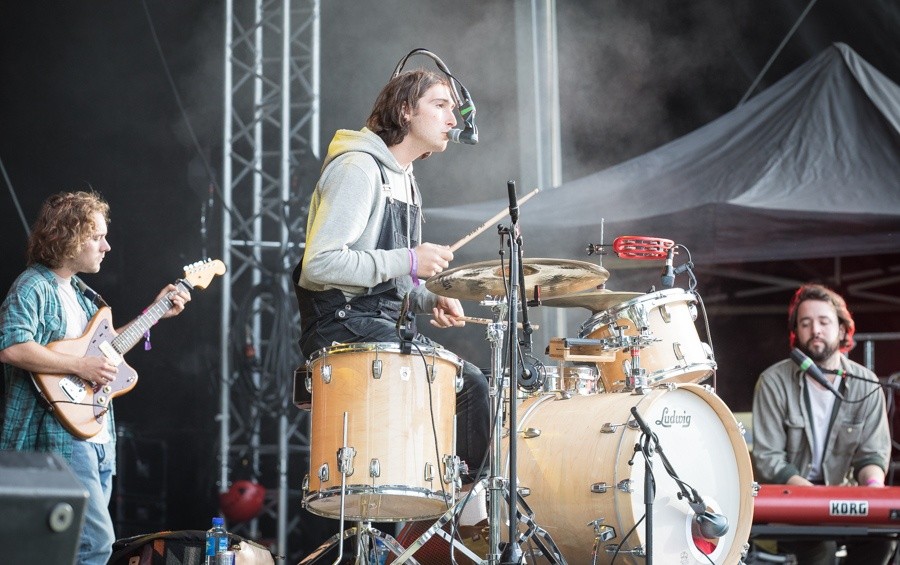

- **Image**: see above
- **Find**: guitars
[28,258,225,439]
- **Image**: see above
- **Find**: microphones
[446,101,479,146]
[660,247,676,287]
[790,348,844,400]
[517,354,547,394]
[692,514,730,539]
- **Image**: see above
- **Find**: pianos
[740,485,900,535]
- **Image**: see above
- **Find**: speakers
[0,450,91,564]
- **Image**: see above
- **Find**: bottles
[206,517,228,565]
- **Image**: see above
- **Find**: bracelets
[136,315,152,351]
[143,309,158,326]
[407,249,420,288]
[864,479,884,487]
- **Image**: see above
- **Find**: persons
[290,70,519,565]
[752,285,897,564]
[0,193,195,565]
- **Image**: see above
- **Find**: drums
[301,341,463,522]
[492,387,759,565]
[578,287,718,395]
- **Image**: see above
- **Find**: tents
[417,40,900,478]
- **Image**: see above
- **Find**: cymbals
[425,257,609,302]
[529,291,647,311]
[613,237,674,261]
